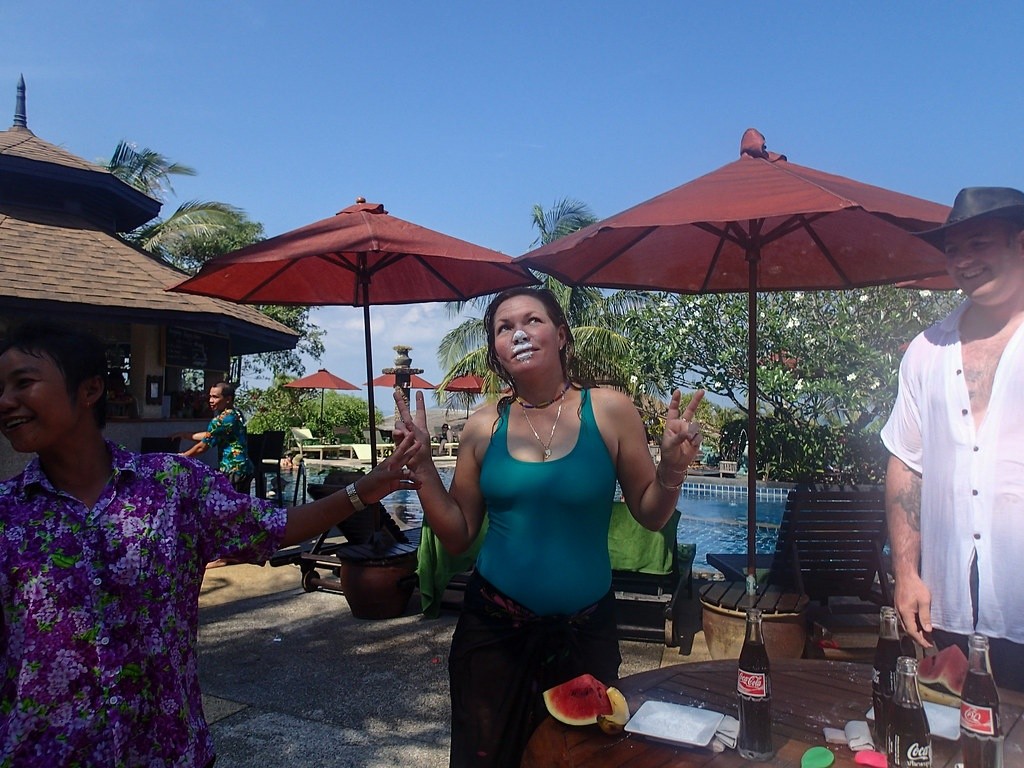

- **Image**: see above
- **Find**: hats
[909,187,1023,254]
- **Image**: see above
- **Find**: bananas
[597,686,630,735]
[918,685,962,710]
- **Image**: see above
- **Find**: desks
[520,649,1024,768]
[335,541,419,618]
[697,582,810,663]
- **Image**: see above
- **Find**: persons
[0,315,422,768]
[169,380,254,568]
[391,286,705,768]
[279,450,309,474]
[879,186,1024,693]
[431,423,465,455]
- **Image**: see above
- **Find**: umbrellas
[365,373,438,390]
[514,127,953,599]
[167,197,546,469]
[444,370,484,420]
[281,368,361,423]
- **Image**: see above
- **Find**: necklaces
[519,382,567,458]
[514,379,572,408]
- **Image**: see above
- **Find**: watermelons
[914,645,967,698]
[543,674,612,730]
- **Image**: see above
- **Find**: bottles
[746,568,756,595]
[885,656,932,768]
[960,634,1004,768]
[873,605,903,752]
[737,608,773,761]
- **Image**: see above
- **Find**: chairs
[299,469,422,592]
[288,425,459,461]
[607,499,696,648]
[705,482,895,664]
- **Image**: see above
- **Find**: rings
[681,416,692,424]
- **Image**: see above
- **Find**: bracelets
[656,468,687,491]
[659,459,689,474]
[345,482,366,513]
